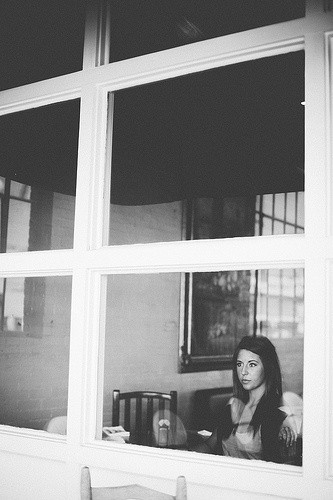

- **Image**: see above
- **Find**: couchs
[193,386,236,439]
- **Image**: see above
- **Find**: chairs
[111,387,178,432]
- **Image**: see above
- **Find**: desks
[125,427,222,456]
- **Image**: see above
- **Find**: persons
[190,334,302,467]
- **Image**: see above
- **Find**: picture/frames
[176,189,262,376]
